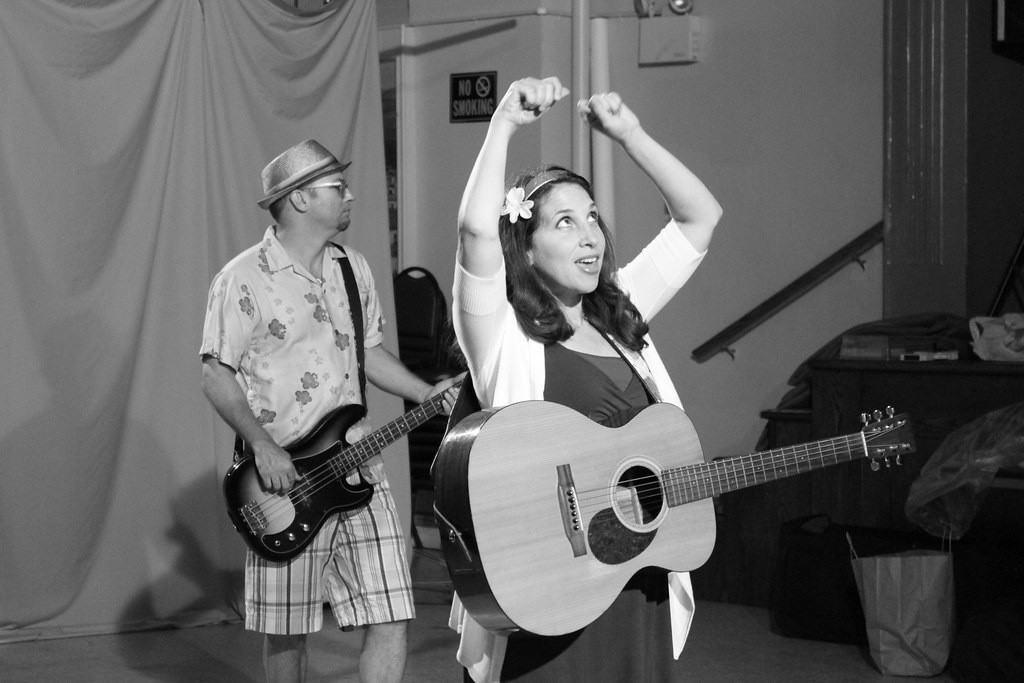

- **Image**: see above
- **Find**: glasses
[296,179,348,193]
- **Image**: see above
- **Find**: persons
[199,136,472,683]
[449,74,725,683]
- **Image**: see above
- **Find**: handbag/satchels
[846,522,955,677]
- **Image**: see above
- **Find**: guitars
[434,399,917,638]
[222,379,464,563]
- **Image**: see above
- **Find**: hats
[257,138,353,210]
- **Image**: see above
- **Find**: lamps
[634,0,701,65]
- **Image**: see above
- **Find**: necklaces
[562,311,583,328]
